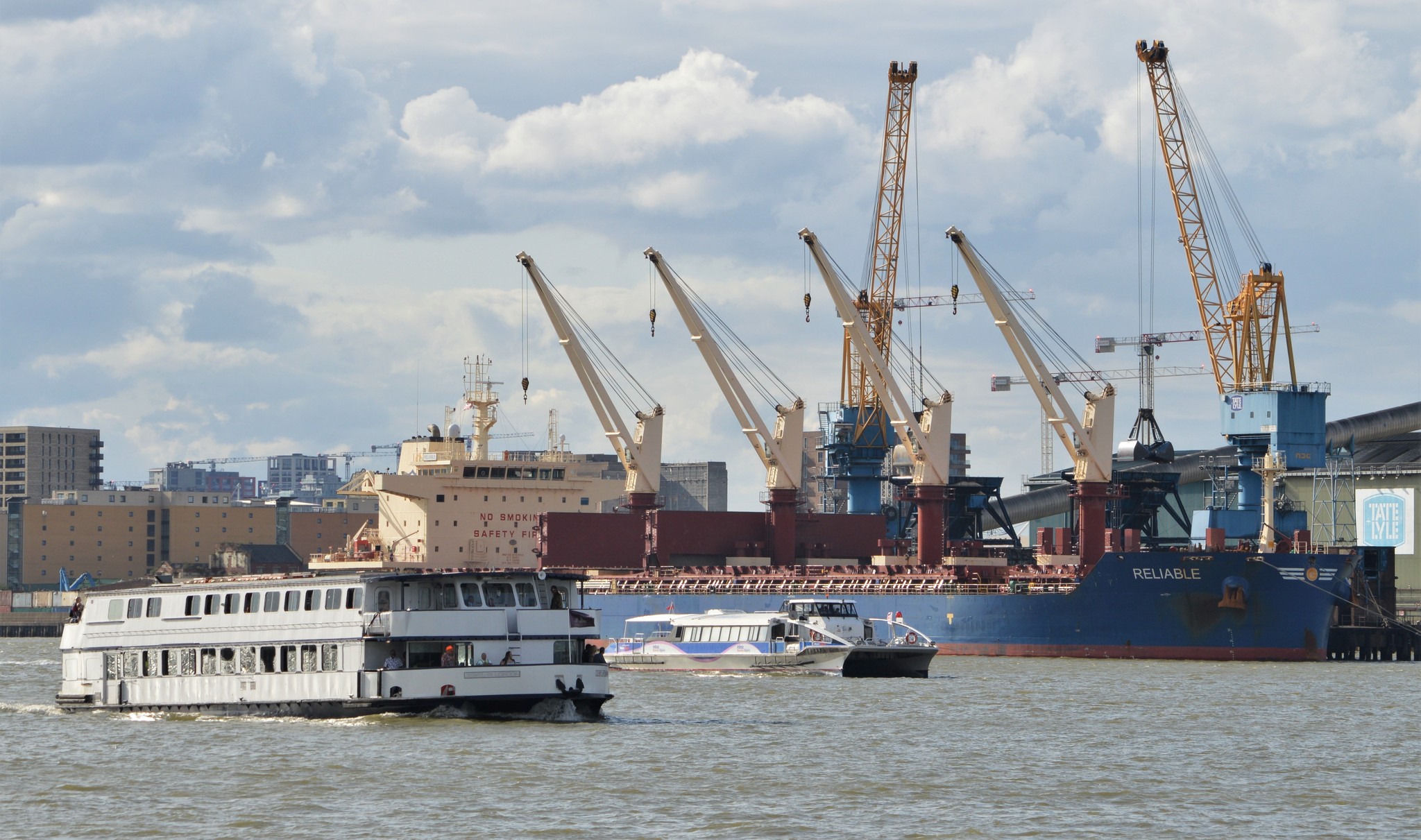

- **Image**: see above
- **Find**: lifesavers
[813,631,823,642]
[906,632,917,644]
[810,629,817,637]
[1000,586,1005,592]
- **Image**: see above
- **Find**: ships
[305,39,1370,663]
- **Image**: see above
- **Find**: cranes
[1136,40,1330,546]
[820,58,969,546]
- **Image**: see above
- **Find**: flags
[666,605,674,610]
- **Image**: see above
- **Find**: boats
[55,568,614,710]
[604,598,939,679]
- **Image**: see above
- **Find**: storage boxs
[532,513,886,568]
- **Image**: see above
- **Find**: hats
[446,646,452,651]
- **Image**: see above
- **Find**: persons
[441,646,459,667]
[550,585,563,610]
[466,596,482,607]
[477,653,493,666]
[819,606,840,614]
[500,651,516,665]
[69,597,84,620]
[582,644,610,666]
[383,650,403,670]
[496,588,509,607]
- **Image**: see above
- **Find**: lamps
[931,641,935,646]
[856,640,861,645]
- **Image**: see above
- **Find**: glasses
[391,654,396,656]
[551,589,555,591]
